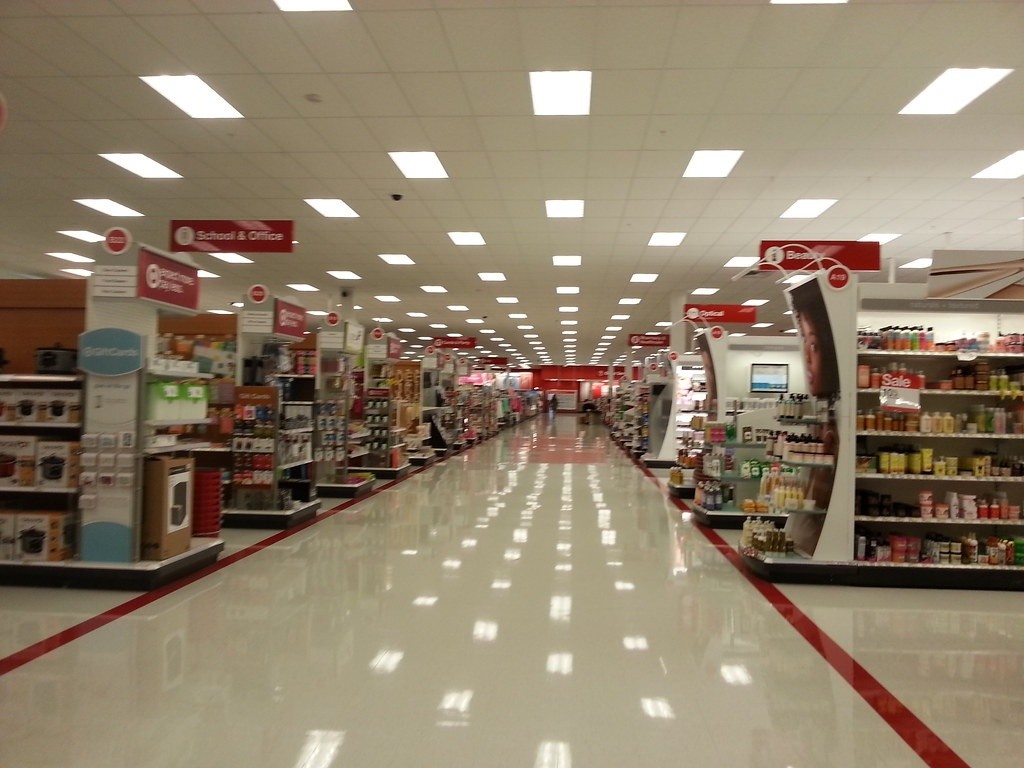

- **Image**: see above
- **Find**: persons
[583,399,595,421]
[788,277,839,396]
[699,351,717,411]
[550,395,558,418]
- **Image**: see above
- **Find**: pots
[38,454,65,480]
[48,400,65,417]
[1,453,16,477]
[17,400,33,415]
[19,530,44,553]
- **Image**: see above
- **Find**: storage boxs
[278,433,313,465]
[274,373,316,405]
[0,513,76,562]
[0,435,84,488]
[280,476,316,503]
[147,383,208,420]
[0,388,84,423]
[192,346,236,375]
[144,459,194,559]
[279,404,313,432]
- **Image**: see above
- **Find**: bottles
[879,326,935,352]
[919,410,954,435]
[870,361,927,391]
[669,398,826,558]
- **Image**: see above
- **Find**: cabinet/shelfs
[161,295,323,529]
[407,349,544,465]
[316,321,375,496]
[0,243,225,589]
[592,261,1024,583]
[349,348,412,479]
